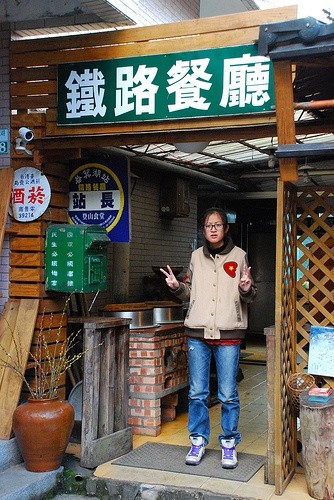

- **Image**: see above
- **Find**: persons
[160,208,258,468]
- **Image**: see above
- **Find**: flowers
[0,289,104,399]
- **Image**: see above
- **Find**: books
[309,388,332,397]
[308,326,334,377]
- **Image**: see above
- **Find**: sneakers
[185,436,205,465]
[220,438,238,468]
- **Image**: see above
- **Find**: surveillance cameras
[15,127,35,151]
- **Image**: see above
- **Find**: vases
[11,397,75,474]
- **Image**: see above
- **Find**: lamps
[260,137,278,167]
[174,142,209,153]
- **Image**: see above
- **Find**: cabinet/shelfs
[160,176,191,217]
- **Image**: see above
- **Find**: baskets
[288,373,315,409]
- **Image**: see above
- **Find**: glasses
[205,224,223,230]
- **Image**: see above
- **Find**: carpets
[111,442,267,482]
[240,349,266,361]
